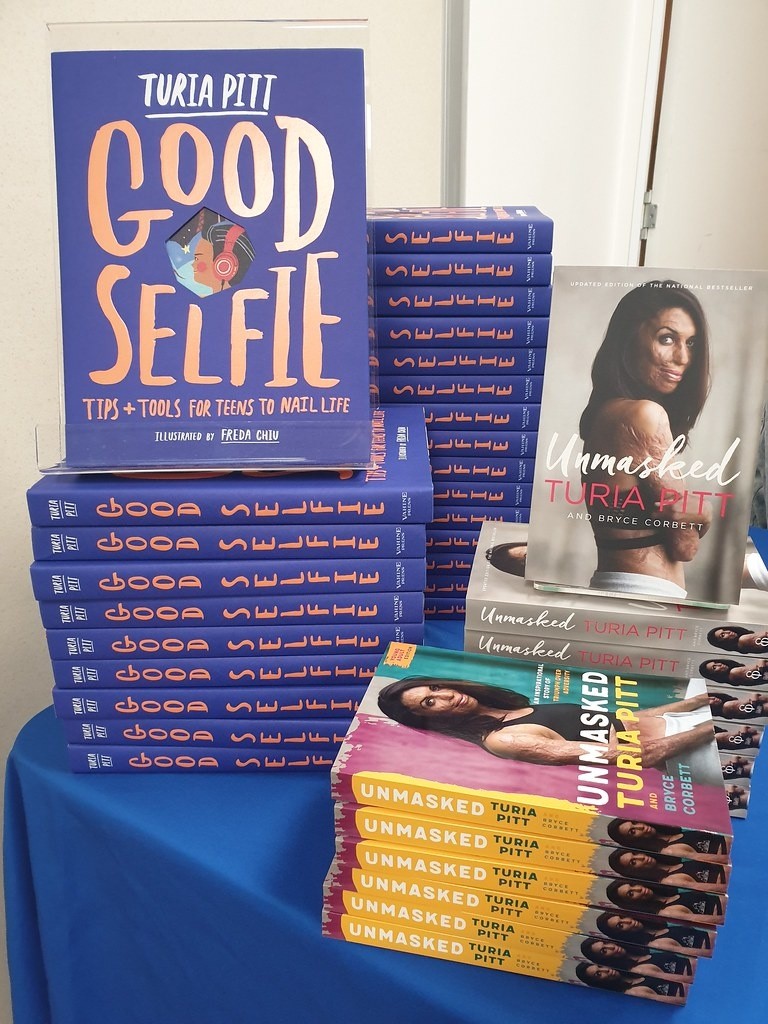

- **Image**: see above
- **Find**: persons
[726,785,750,810]
[607,818,733,865]
[607,879,726,925]
[581,938,696,984]
[609,848,730,894]
[721,755,754,781]
[596,912,715,958]
[706,626,768,654]
[579,281,713,598]
[376,675,725,787]
[576,962,689,1006]
[699,659,768,686]
[707,692,768,719]
[714,726,764,750]
[487,543,768,591]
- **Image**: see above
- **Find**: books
[27,45,554,777]
[465,264,768,820]
[321,640,733,1008]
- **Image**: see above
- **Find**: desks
[0,698,768,1024]
